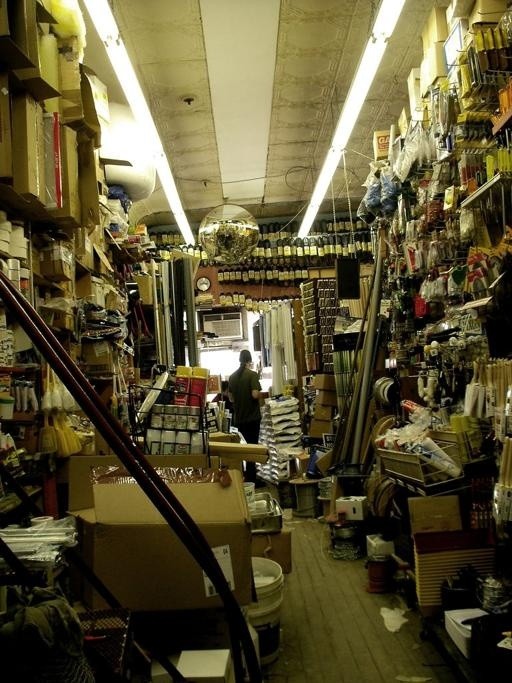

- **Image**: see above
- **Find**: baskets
[376,438,466,487]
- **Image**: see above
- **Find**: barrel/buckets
[245,556,285,665]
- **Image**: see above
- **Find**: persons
[226,349,269,488]
[211,380,234,411]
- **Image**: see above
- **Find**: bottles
[149,217,374,310]
